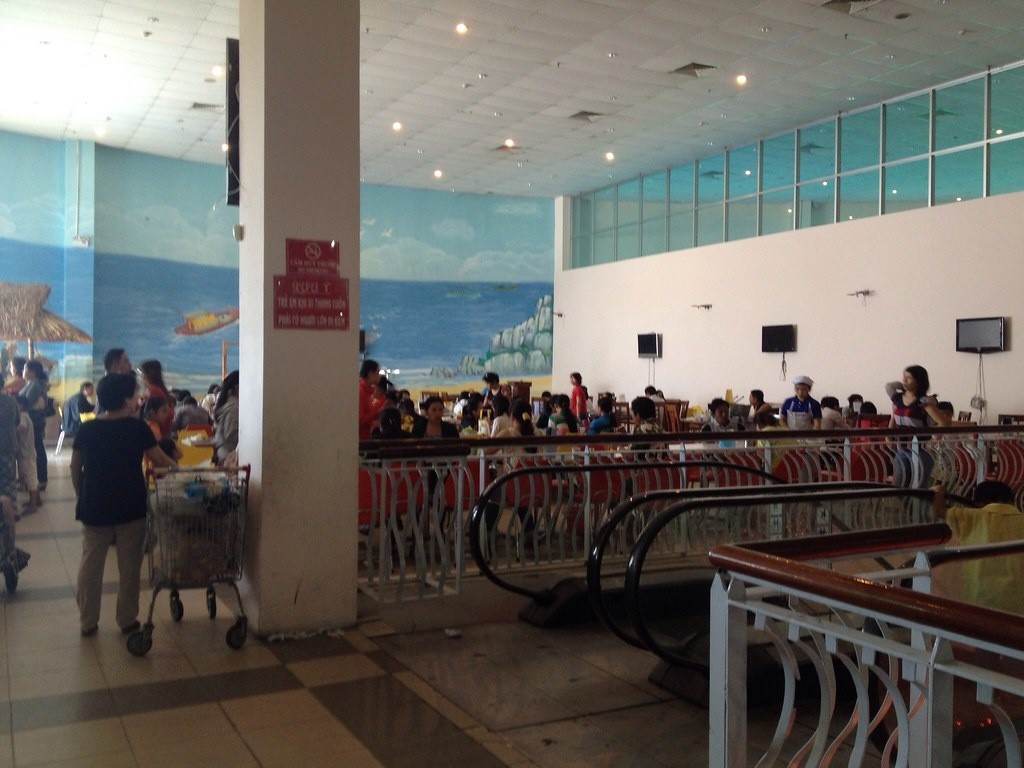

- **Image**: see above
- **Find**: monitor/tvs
[638,334,658,358]
[762,325,794,353]
[956,317,1004,353]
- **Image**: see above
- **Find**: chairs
[55,406,65,455]
[185,424,213,436]
[357,393,1023,566]
[176,430,214,467]
[79,412,95,423]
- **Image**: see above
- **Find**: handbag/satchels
[23,382,56,418]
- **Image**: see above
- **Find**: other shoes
[21,508,36,516]
[16,548,31,571]
[21,500,42,506]
[4,550,18,594]
[122,620,140,632]
[82,625,98,635]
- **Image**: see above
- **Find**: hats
[791,375,814,387]
[483,372,498,381]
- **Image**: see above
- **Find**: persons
[929,481,1024,618]
[0,347,955,633]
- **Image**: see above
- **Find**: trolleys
[125,460,253,654]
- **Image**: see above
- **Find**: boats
[176,307,240,337]
